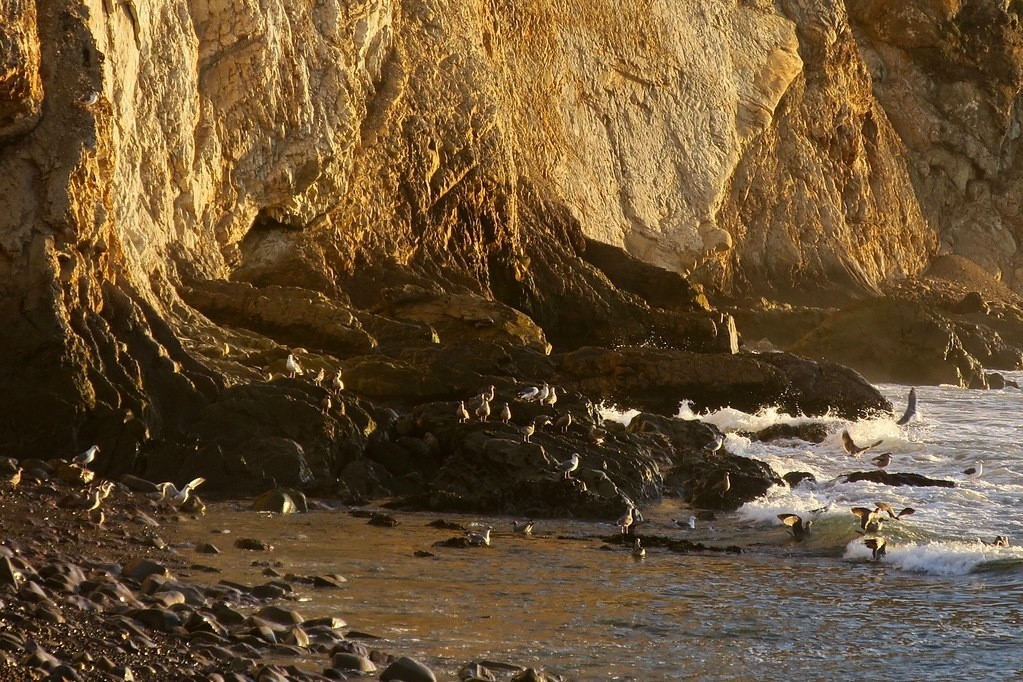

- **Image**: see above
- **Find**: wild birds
[60,353,990,564]
